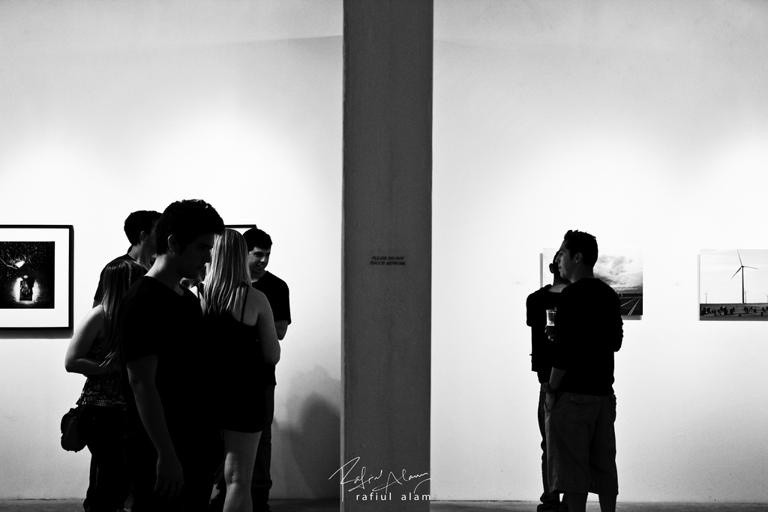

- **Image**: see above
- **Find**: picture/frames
[0,225,74,332]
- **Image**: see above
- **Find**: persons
[64,259,155,510]
[524,251,568,512]
[91,207,163,309]
[240,227,292,510]
[117,197,228,509]
[543,229,620,511]
[187,228,282,510]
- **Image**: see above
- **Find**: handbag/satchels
[60,404,92,452]
